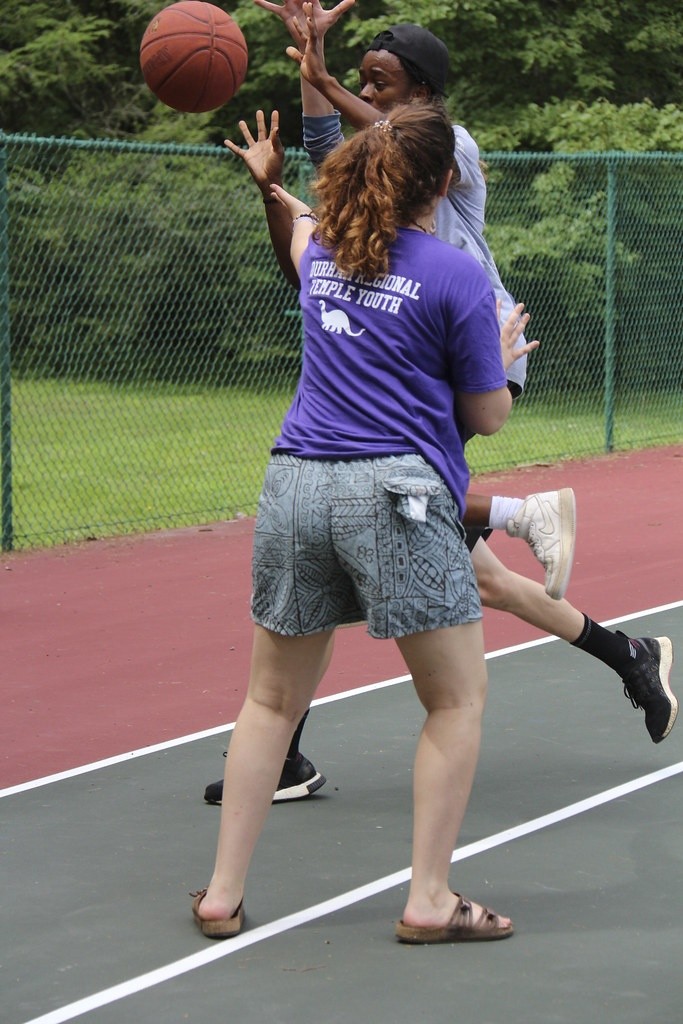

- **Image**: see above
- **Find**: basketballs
[139,0,248,113]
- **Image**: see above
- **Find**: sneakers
[613,630,679,745]
[204,751,326,805]
[507,488,577,601]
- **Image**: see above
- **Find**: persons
[197,11,678,814]
[195,102,542,945]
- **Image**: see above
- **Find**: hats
[365,24,451,99]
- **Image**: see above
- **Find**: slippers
[189,885,243,936]
[396,892,513,944]
